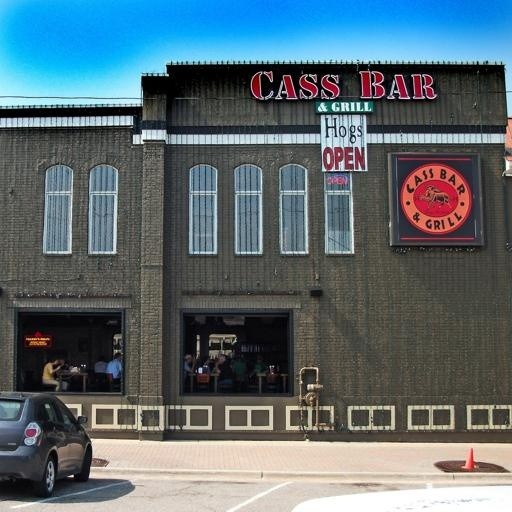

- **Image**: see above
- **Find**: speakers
[309,289,323,297]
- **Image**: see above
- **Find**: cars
[0,391,93,498]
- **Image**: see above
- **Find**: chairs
[96,372,119,391]
[29,368,56,392]
[219,362,245,392]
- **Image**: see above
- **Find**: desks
[187,372,219,393]
[255,372,289,395]
[55,371,88,391]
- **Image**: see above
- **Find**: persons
[239,353,247,376]
[253,354,266,373]
[106,352,121,383]
[214,354,234,385]
[42,359,62,391]
[56,359,70,391]
[93,355,108,373]
[184,354,197,378]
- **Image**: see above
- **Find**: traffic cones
[461,447,479,470]
